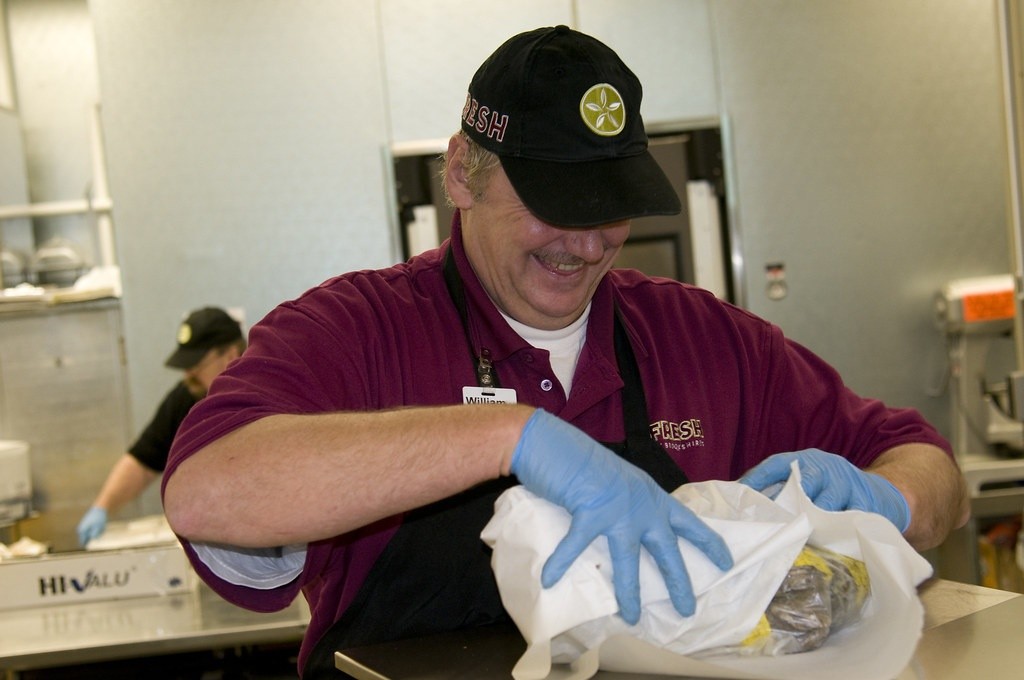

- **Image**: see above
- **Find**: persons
[75,306,249,543]
[158,25,971,680]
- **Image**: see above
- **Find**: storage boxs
[0,515,204,612]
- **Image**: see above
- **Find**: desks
[1,583,314,680]
[334,578,1024,679]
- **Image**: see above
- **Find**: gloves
[76,505,108,547]
[738,448,911,534]
[510,408,734,626]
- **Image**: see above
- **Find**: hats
[166,308,241,369]
[461,26,683,227]
